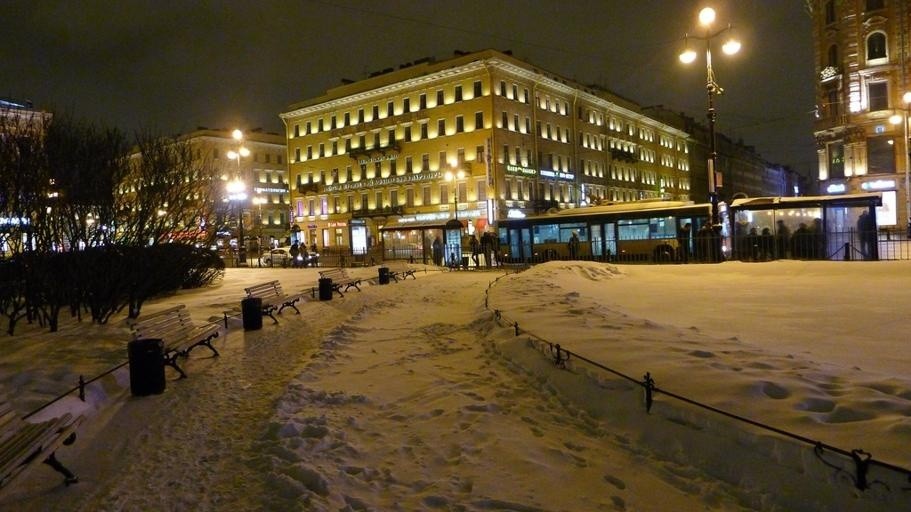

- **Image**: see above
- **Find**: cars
[263,245,320,268]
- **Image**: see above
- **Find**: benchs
[122,260,416,383]
[1,381,88,501]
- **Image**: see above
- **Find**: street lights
[889,91,911,240]
[227,129,253,267]
[678,7,747,263]
[445,160,466,219]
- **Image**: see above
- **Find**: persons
[311,243,319,268]
[856,210,874,259]
[468,232,505,269]
[424,236,433,260]
[298,242,309,269]
[290,240,299,268]
[677,217,823,263]
[568,231,579,260]
[432,235,443,267]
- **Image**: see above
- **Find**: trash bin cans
[378,268,389,285]
[319,278,332,300]
[241,298,262,330]
[127,338,166,397]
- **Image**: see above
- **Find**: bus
[490,199,717,264]
